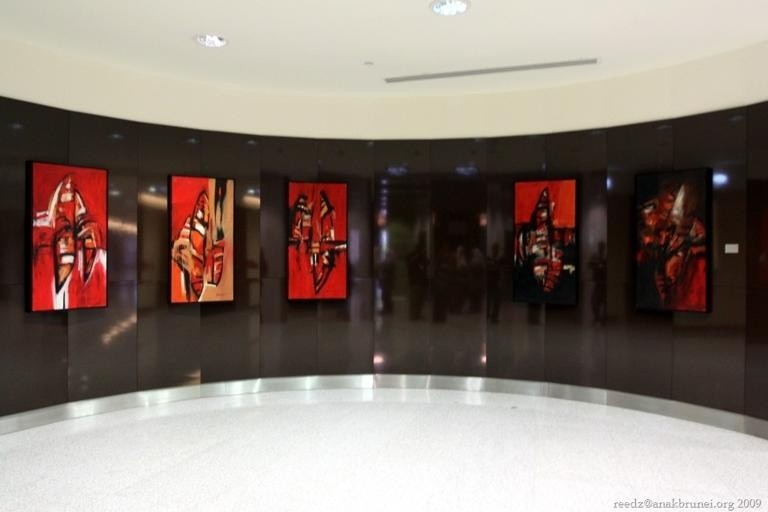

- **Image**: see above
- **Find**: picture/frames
[633,166,713,315]
[166,174,237,305]
[24,160,108,313]
